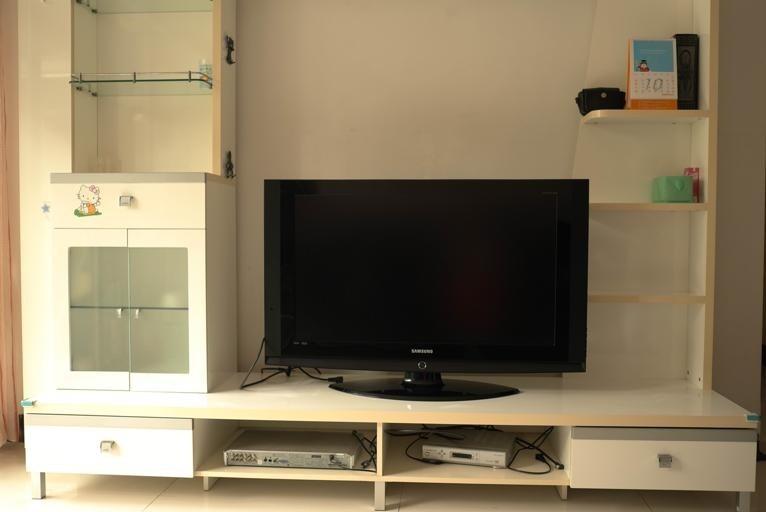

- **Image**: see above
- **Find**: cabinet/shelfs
[23,374,760,510]
[49,172,240,393]
[572,107,717,390]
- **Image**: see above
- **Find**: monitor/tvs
[263,179,590,402]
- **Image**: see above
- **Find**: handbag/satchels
[574,85,627,119]
